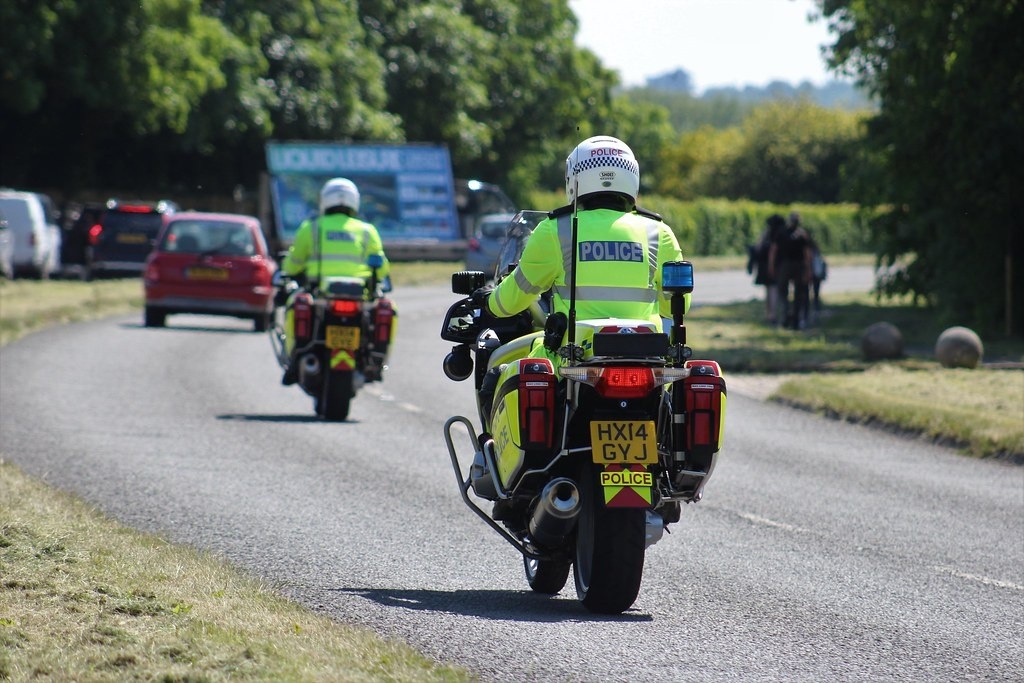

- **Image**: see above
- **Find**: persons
[469,133,691,519]
[753,212,826,330]
[278,175,393,387]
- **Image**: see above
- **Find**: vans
[463,209,554,281]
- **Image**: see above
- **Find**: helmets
[320,176,360,215]
[565,135,639,210]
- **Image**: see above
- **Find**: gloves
[472,285,498,319]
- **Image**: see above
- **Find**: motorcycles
[264,248,399,425]
[438,209,728,618]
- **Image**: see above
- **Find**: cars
[83,197,183,279]
[0,188,66,281]
[140,211,283,332]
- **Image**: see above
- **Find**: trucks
[255,141,523,263]
